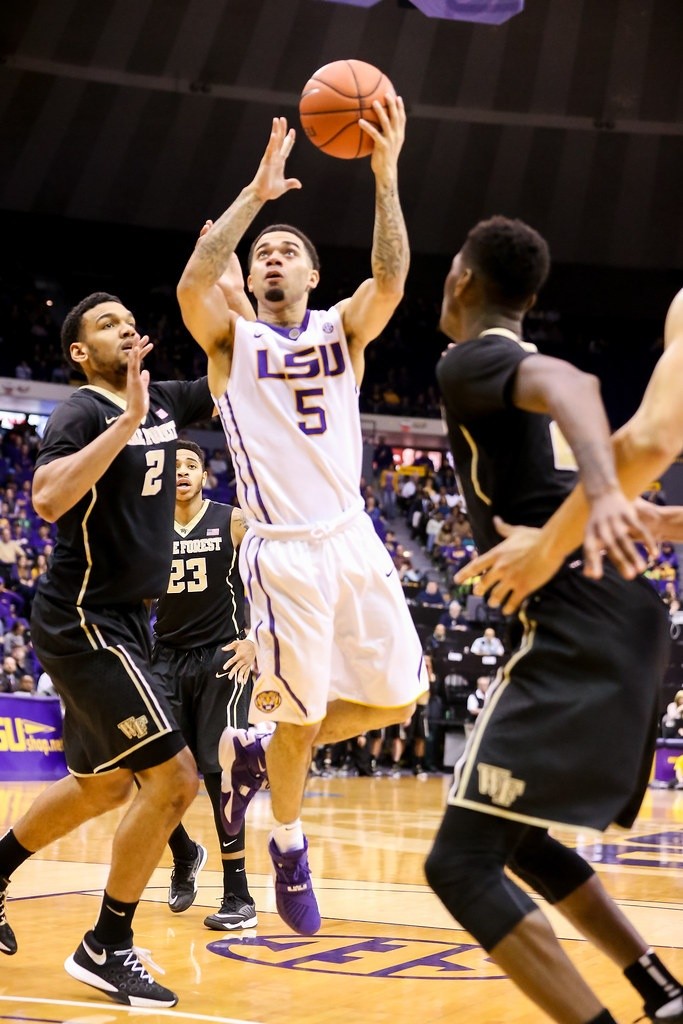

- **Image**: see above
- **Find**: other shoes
[642,986,683,1024]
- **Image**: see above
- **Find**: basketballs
[298,60,397,160]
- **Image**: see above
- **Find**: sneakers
[0,878,17,956]
[218,724,270,836]
[203,892,258,931]
[167,840,207,913]
[64,930,179,1008]
[268,829,321,936]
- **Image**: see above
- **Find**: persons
[467,675,491,723]
[438,598,472,632]
[444,536,505,622]
[666,690,683,740]
[372,433,397,475]
[0,418,66,717]
[176,92,431,939]
[359,478,426,583]
[415,581,447,608]
[423,213,683,1024]
[394,448,473,560]
[199,439,240,506]
[424,624,457,652]
[639,541,683,614]
[0,219,257,1009]
[469,627,505,656]
[309,653,441,781]
